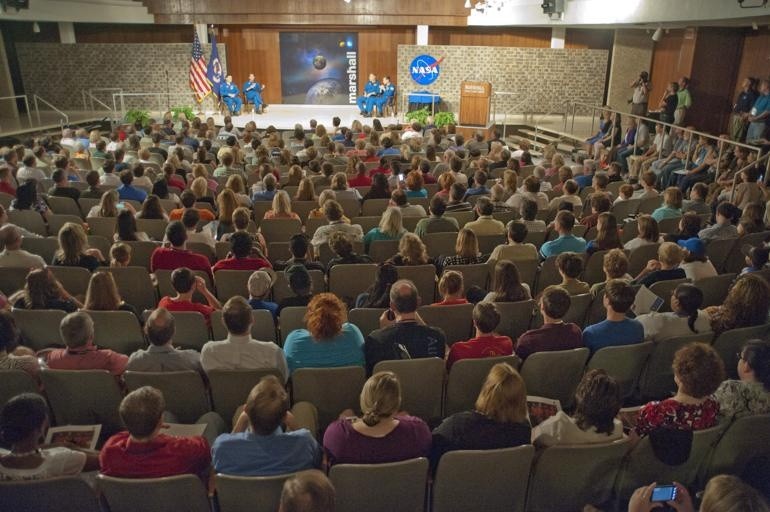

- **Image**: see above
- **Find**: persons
[630,388,633,389]
[629,72,692,134]
[629,342,727,453]
[220,73,268,115]
[276,469,337,512]
[430,363,531,479]
[0,392,100,482]
[1,107,770,387]
[98,386,229,490]
[628,475,770,512]
[531,367,624,463]
[210,375,323,477]
[323,371,433,466]
[357,73,395,117]
[732,78,770,143]
[710,338,770,425]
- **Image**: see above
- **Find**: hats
[248,267,278,297]
[284,264,312,286]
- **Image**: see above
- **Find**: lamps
[32,22,40,34]
[651,26,664,43]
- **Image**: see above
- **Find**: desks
[403,90,440,116]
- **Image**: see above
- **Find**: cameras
[650,484,678,502]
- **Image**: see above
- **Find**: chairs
[245,93,262,114]
[363,94,377,117]
[375,90,397,117]
[221,94,243,117]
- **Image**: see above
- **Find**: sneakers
[256,110,261,114]
[376,113,383,117]
[360,111,372,117]
[230,111,238,116]
[263,103,268,107]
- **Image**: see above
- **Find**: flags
[205,34,224,89]
[188,31,212,104]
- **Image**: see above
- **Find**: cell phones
[399,173,403,182]
